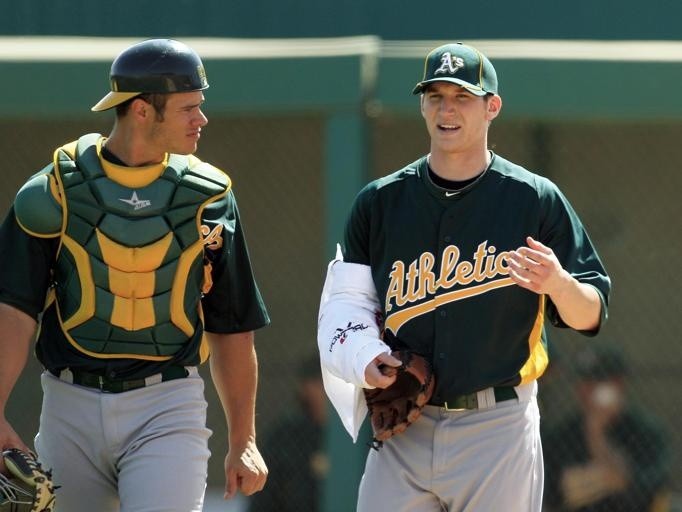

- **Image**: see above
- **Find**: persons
[0,39,270,512]
[316,43,611,512]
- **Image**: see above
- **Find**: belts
[55,367,190,393]
[427,387,517,412]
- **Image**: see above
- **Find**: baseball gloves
[363,349,434,440]
[0,447,57,512]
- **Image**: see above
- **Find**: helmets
[90,37,211,113]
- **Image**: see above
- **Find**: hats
[412,42,498,98]
